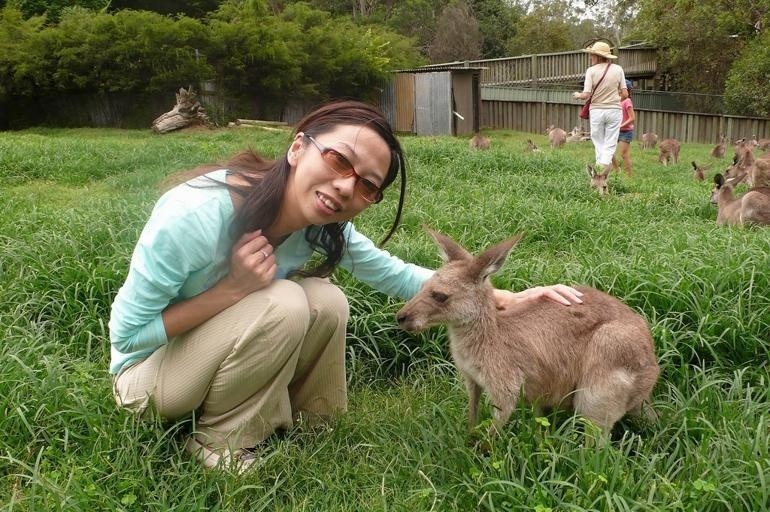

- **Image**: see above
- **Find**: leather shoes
[186,428,267,480]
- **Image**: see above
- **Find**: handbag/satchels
[578,96,591,119]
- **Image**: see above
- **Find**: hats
[625,78,633,90]
[580,41,619,60]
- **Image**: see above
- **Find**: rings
[259,249,267,259]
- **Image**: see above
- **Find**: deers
[640,127,657,151]
[394,221,662,452]
[525,139,545,155]
[470,134,491,151]
[658,138,681,168]
[545,122,592,147]
[692,132,769,229]
[585,162,665,205]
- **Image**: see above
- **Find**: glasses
[304,134,384,205]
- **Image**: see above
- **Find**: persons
[612,79,635,175]
[107,101,585,475]
[573,41,629,173]
